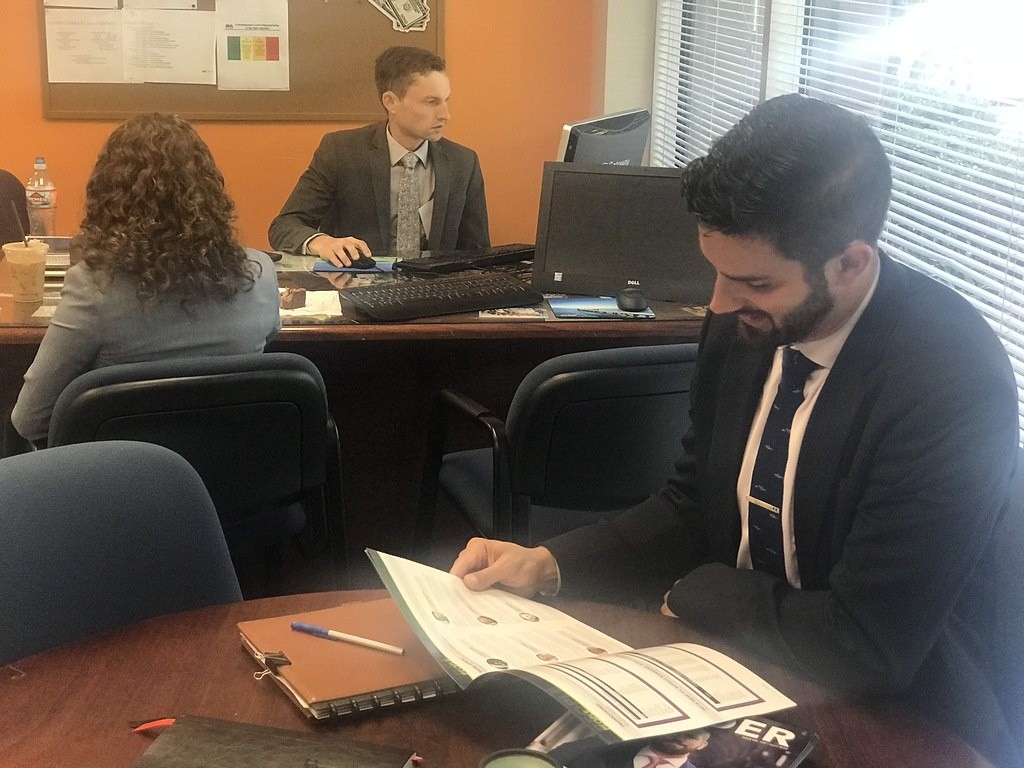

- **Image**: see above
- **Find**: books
[524,710,821,768]
[136,715,422,767]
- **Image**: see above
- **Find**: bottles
[24,157,57,237]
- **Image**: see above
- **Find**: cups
[477,748,561,768]
[2,242,49,302]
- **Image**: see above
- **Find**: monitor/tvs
[556,106,651,167]
[530,160,718,307]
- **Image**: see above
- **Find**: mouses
[616,287,648,313]
[329,249,376,270]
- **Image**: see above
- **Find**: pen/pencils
[291,621,406,656]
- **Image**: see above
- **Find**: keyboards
[391,243,536,275]
[337,271,544,323]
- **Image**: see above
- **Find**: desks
[0,231,714,344]
[0,590,996,768]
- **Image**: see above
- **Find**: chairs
[422,343,705,553]
[1,441,248,669]
[50,354,344,609]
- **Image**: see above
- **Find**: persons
[268,47,492,268]
[0,169,30,251]
[446,94,1024,768]
[574,728,711,768]
[11,111,282,441]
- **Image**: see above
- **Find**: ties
[748,348,824,585]
[644,754,668,768]
[397,152,422,260]
